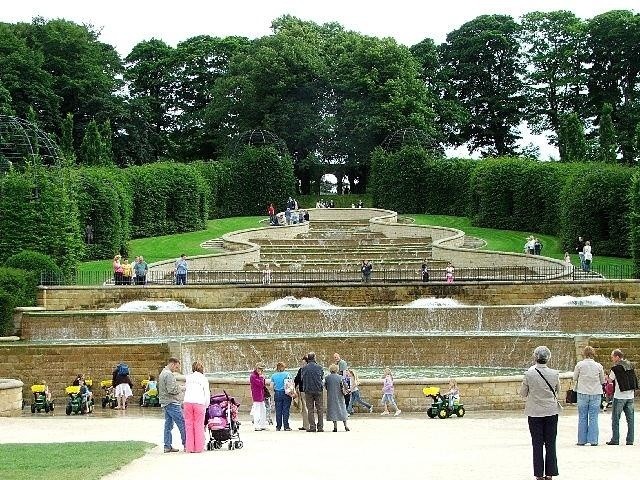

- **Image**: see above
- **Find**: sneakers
[576,441,634,446]
[253,426,350,433]
[163,446,180,453]
[114,406,125,410]
[393,409,401,417]
[535,475,553,480]
[368,404,374,413]
[380,410,391,416]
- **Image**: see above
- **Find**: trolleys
[600,382,613,410]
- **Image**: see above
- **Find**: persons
[380,367,402,417]
[519,345,563,478]
[582,240,593,272]
[134,255,148,285]
[575,237,585,270]
[248,350,374,435]
[573,346,604,446]
[121,259,134,285]
[604,348,636,446]
[267,195,363,224]
[158,357,187,454]
[529,235,535,255]
[173,253,190,285]
[534,238,543,256]
[183,361,212,453]
[524,236,530,254]
[443,378,459,410]
[130,256,139,284]
[564,251,571,263]
[362,259,373,283]
[422,262,429,282]
[261,264,273,285]
[65,362,162,416]
[445,260,455,283]
[113,255,123,285]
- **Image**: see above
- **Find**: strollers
[207,389,245,451]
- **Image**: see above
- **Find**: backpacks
[116,363,130,377]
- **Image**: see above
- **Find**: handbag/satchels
[602,380,615,396]
[564,377,578,404]
